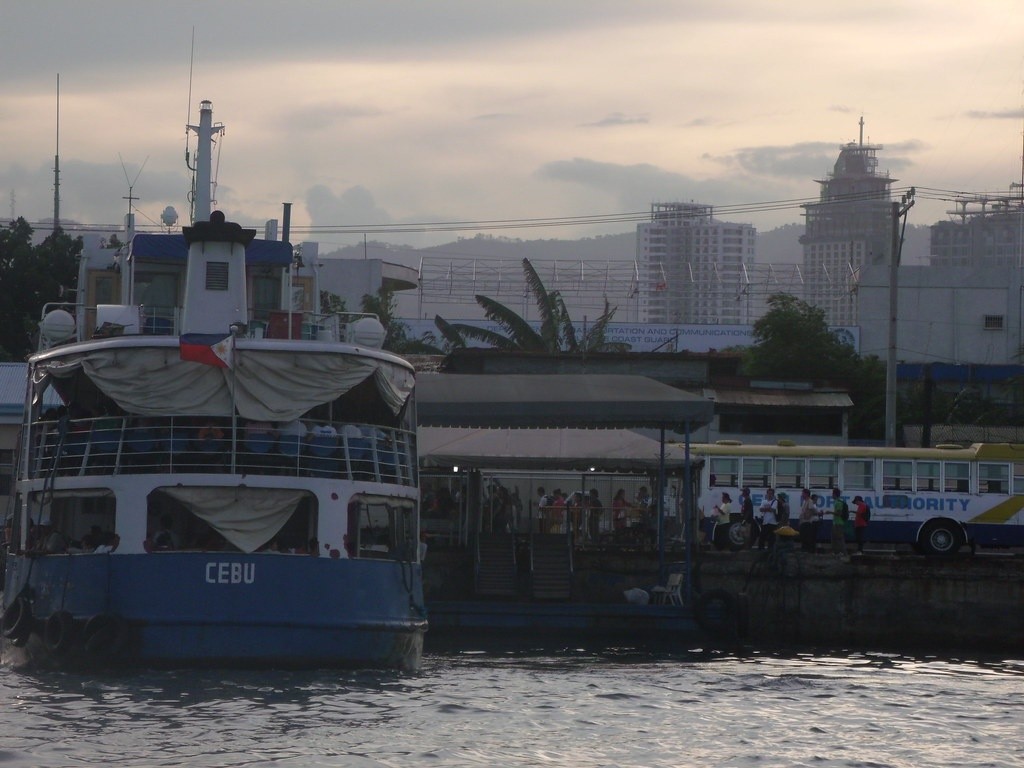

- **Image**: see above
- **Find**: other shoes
[832,552,844,557]
[853,551,864,556]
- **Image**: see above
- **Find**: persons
[37,404,417,483]
[422,481,871,555]
[0,516,318,557]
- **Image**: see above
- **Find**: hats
[852,496,862,504]
[777,492,786,499]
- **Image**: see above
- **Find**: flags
[179,332,237,374]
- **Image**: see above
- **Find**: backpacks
[838,499,849,522]
[861,503,870,522]
[771,499,783,522]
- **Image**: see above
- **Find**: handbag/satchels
[550,524,560,534]
[612,498,626,520]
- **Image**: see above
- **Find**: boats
[417,373,715,633]
[3,95,431,675]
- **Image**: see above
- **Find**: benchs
[650,573,684,606]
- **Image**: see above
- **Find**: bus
[677,439,1024,561]
